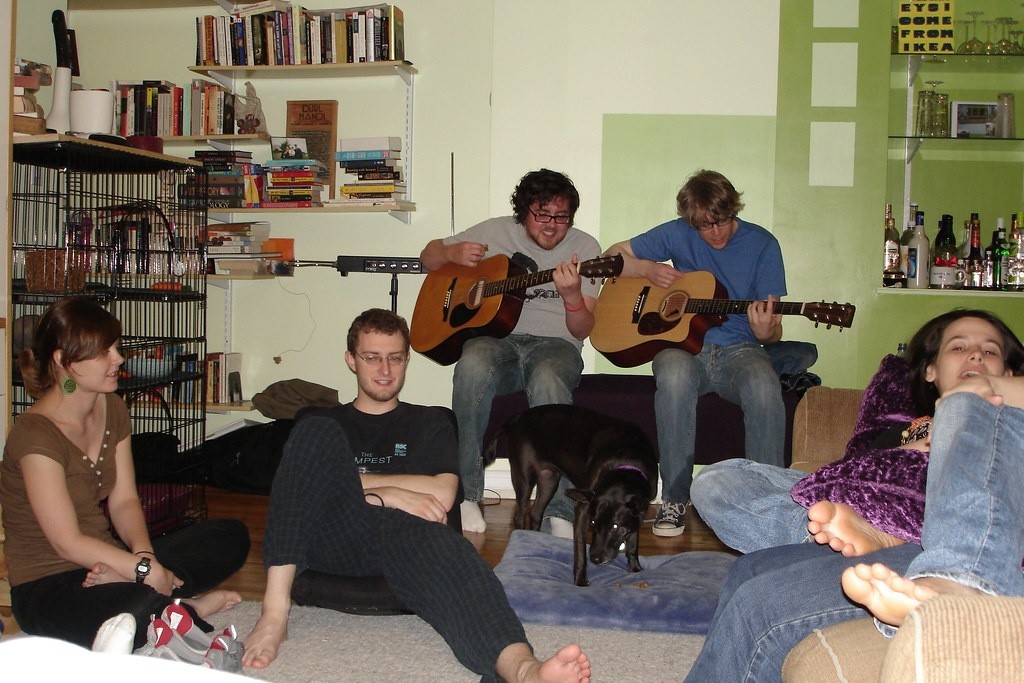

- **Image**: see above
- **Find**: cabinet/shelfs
[876,0,1024,299]
[86,60,419,415]
[10,133,212,539]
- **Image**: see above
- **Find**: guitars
[587,269,858,370]
[408,252,624,366]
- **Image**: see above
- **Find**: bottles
[883,203,1024,292]
[895,343,908,359]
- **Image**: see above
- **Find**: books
[187,150,266,209]
[109,79,236,137]
[261,160,327,208]
[200,220,271,274]
[13,64,53,137]
[323,136,416,206]
[61,207,208,275]
[122,351,241,403]
[193,0,405,65]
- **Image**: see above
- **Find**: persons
[0,296,252,654]
[600,167,787,539]
[682,306,1024,683]
[419,169,606,533]
[242,307,592,683]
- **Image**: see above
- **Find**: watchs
[135,557,153,585]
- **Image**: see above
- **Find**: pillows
[492,529,738,634]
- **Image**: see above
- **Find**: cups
[915,91,948,138]
[25,249,87,293]
[69,90,114,138]
[998,93,1014,139]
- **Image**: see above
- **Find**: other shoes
[134,599,244,673]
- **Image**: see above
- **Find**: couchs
[780,385,1024,683]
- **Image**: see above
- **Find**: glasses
[527,206,571,224]
[355,349,406,366]
[693,215,734,231]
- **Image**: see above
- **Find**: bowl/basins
[123,359,177,379]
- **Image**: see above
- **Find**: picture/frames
[270,136,310,160]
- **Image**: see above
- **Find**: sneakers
[652,499,690,536]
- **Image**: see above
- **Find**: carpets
[133,595,707,683]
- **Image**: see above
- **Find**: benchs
[481,374,803,506]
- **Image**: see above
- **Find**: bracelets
[135,550,156,556]
[563,298,584,312]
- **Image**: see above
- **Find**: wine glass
[955,11,1024,54]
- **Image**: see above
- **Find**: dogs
[483,405,658,585]
